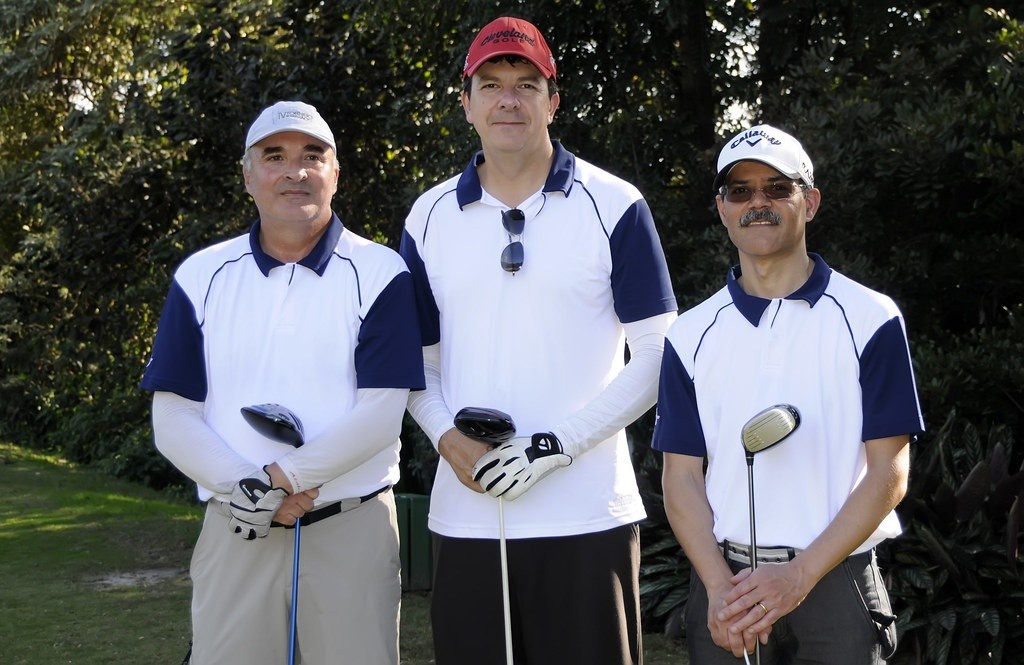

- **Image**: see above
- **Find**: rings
[759,603,767,615]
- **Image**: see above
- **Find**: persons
[651,123,926,665]
[399,17,679,665]
[140,101,426,665]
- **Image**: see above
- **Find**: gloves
[215,465,290,540]
[471,431,572,501]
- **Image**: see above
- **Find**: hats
[462,17,556,83]
[713,124,814,191]
[245,101,336,159]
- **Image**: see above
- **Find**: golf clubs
[451,405,516,665]
[240,400,303,664]
[740,402,803,664]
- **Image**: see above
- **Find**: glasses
[718,182,806,204]
[501,206,525,277]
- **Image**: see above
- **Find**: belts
[221,485,390,530]
[717,539,804,568]
